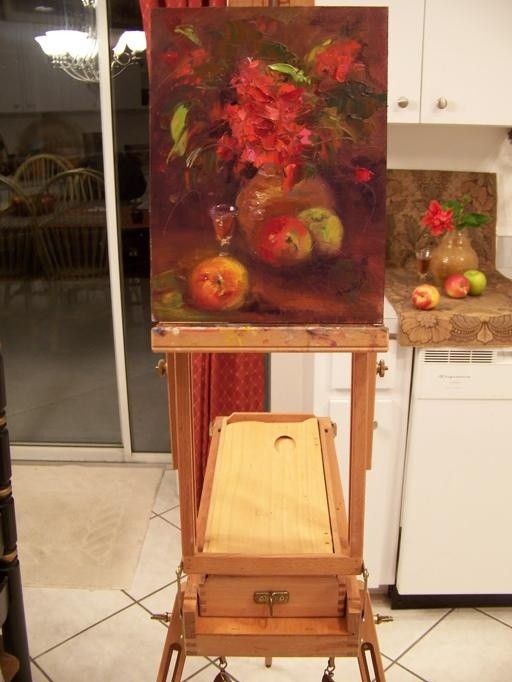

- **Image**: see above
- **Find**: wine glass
[416,249,433,288]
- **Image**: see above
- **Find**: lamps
[34,1,147,80]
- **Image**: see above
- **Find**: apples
[256,209,344,269]
[413,285,438,312]
[189,253,251,314]
[446,269,487,299]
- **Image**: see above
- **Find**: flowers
[422,194,482,238]
[184,30,378,186]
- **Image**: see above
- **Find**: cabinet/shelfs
[148,321,389,682]
[389,348,512,609]
[387,0,512,125]
[270,339,414,596]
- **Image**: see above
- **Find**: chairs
[4,115,106,277]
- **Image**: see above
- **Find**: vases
[236,168,341,271]
[424,233,480,292]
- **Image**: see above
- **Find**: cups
[209,204,237,258]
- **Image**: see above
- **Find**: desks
[122,202,148,327]
